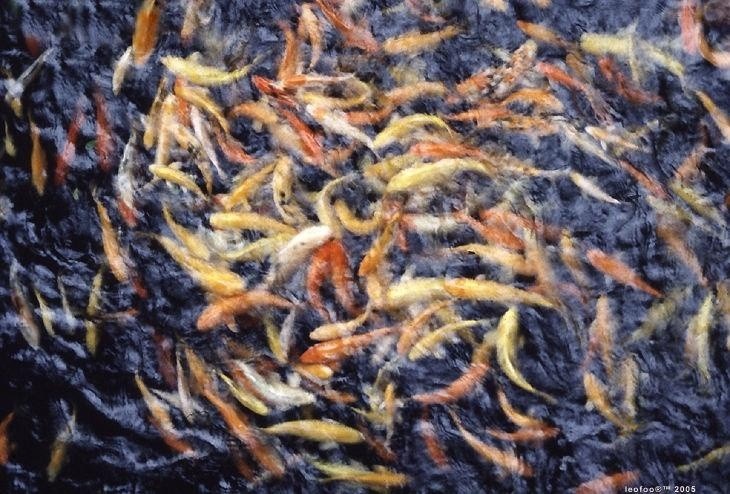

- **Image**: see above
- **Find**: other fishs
[0,0,729,494]
